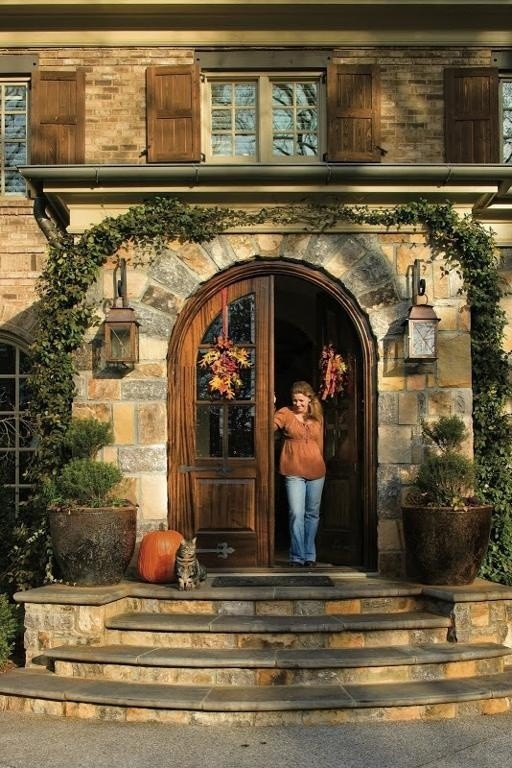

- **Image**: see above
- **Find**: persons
[273,380,327,567]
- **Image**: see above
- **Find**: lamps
[102,258,143,370]
[400,259,442,375]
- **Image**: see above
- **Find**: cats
[173,536,207,591]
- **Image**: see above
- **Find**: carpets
[212,576,335,587]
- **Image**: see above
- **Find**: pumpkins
[135,523,187,584]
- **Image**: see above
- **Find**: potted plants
[42,416,138,588]
[399,413,495,587]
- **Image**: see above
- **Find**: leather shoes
[289,561,302,568]
[304,561,316,568]
